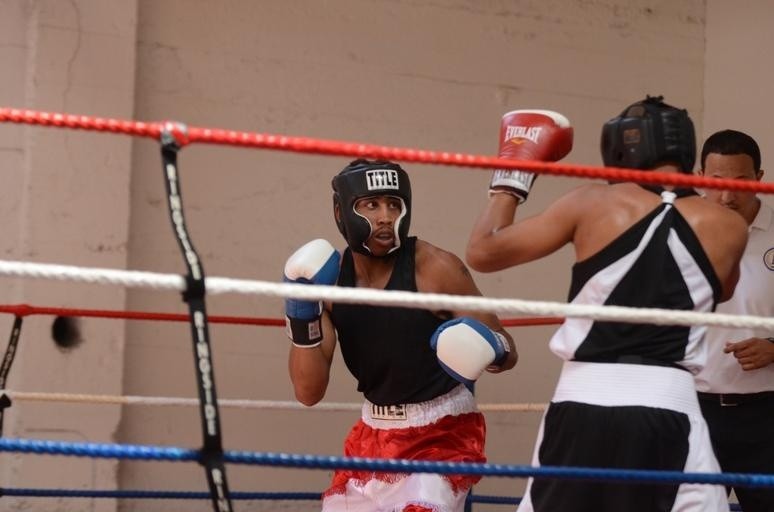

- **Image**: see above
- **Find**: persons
[465,94,752,511]
[695,126,774,512]
[278,156,519,510]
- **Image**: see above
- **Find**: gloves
[429,316,511,385]
[282,238,341,349]
[487,108,574,205]
